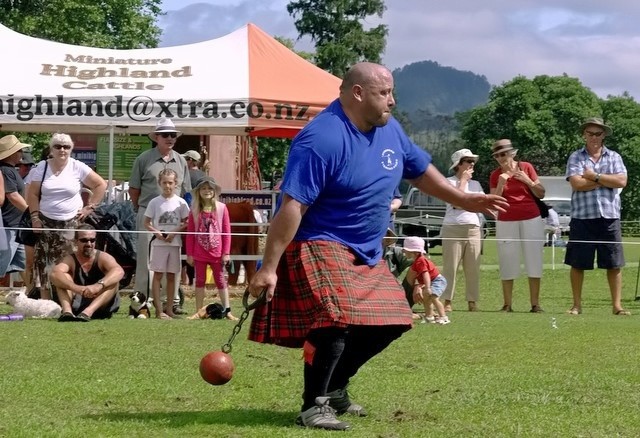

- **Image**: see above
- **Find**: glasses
[76,238,97,243]
[463,159,477,164]
[583,129,605,137]
[52,144,73,150]
[494,151,511,157]
[156,132,179,137]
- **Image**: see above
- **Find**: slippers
[73,313,92,321]
[530,303,543,311]
[567,306,582,314]
[613,307,633,317]
[58,312,75,322]
[499,303,513,313]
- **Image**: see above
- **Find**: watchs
[98,279,106,290]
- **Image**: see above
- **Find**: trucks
[402,184,486,254]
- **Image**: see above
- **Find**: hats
[449,147,480,171]
[0,135,32,159]
[579,116,614,137]
[148,120,183,144]
[179,149,203,164]
[489,139,519,156]
[192,177,221,195]
[401,236,428,255]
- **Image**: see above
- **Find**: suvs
[538,177,573,235]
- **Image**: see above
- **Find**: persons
[178,149,208,288]
[15,151,35,183]
[541,202,560,247]
[128,118,193,315]
[564,117,632,316]
[383,228,426,309]
[400,237,450,325]
[49,223,125,322]
[248,60,509,431]
[441,148,486,312]
[0,169,9,277]
[143,168,192,322]
[489,139,546,313]
[185,176,240,322]
[27,132,108,309]
[384,183,404,233]
[0,134,32,287]
[14,146,54,296]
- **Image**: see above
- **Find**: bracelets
[86,203,96,212]
[595,173,601,182]
[29,210,44,227]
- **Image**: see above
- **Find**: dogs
[187,303,231,319]
[127,291,151,319]
[5,287,62,318]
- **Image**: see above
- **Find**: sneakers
[329,398,368,418]
[293,397,351,431]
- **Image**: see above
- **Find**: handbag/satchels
[518,162,552,219]
[19,160,48,246]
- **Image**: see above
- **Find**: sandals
[420,316,436,322]
[434,317,450,325]
